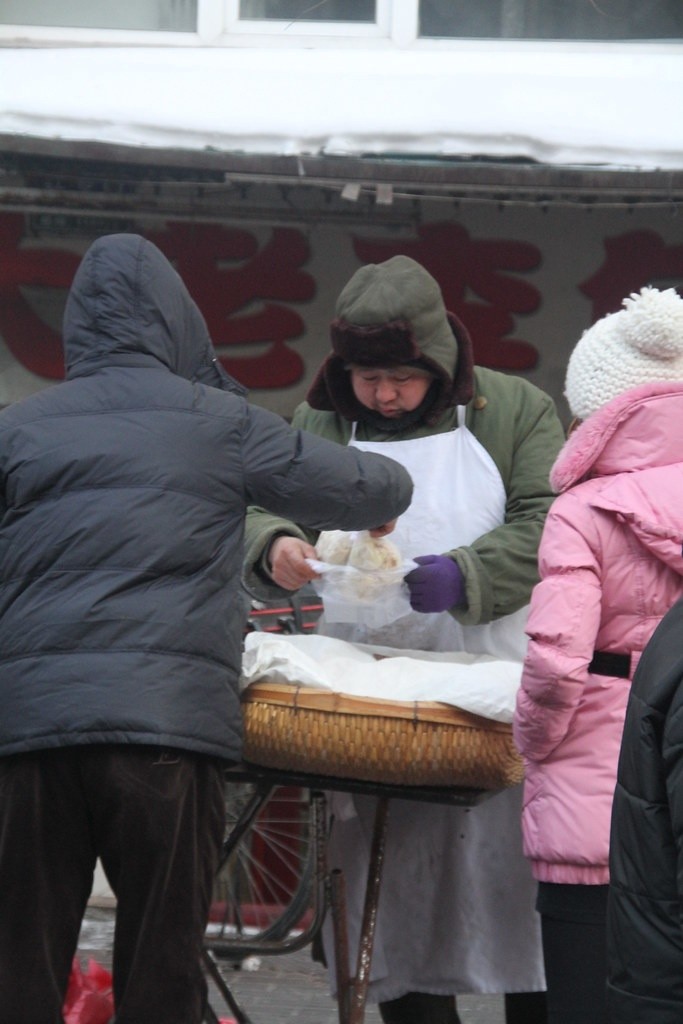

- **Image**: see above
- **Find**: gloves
[406,556,461,613]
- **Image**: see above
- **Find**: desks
[200,763,508,1024]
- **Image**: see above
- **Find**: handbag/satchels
[62,954,115,1024]
[244,597,324,635]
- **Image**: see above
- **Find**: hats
[303,255,475,433]
[563,286,682,420]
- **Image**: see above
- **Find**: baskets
[238,681,524,786]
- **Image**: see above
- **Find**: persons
[1,232,413,1024]
[510,285,683,1024]
[601,598,683,1024]
[238,254,565,1024]
